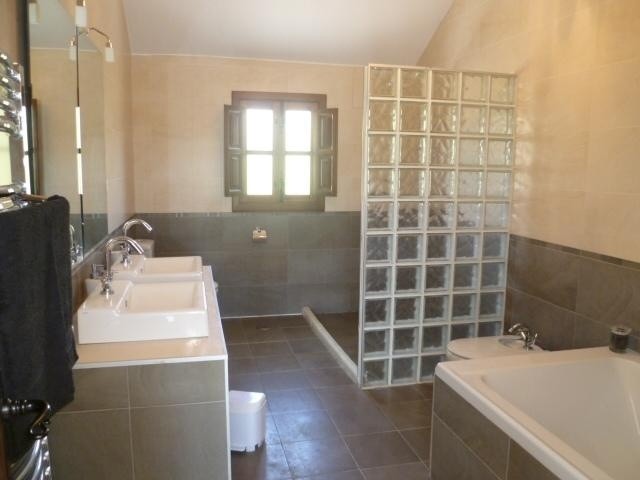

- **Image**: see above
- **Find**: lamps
[28,1,40,24]
[90,28,113,63]
[69,30,87,61]
[76,1,87,28]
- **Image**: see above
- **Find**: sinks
[446,334,542,360]
[111,255,204,280]
[77,280,209,345]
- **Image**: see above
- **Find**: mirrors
[23,1,108,279]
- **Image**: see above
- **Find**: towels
[0,195,79,463]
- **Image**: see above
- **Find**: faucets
[123,218,153,265]
[509,322,539,350]
[70,225,76,253]
[104,237,145,294]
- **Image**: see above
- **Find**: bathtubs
[429,346,640,479]
